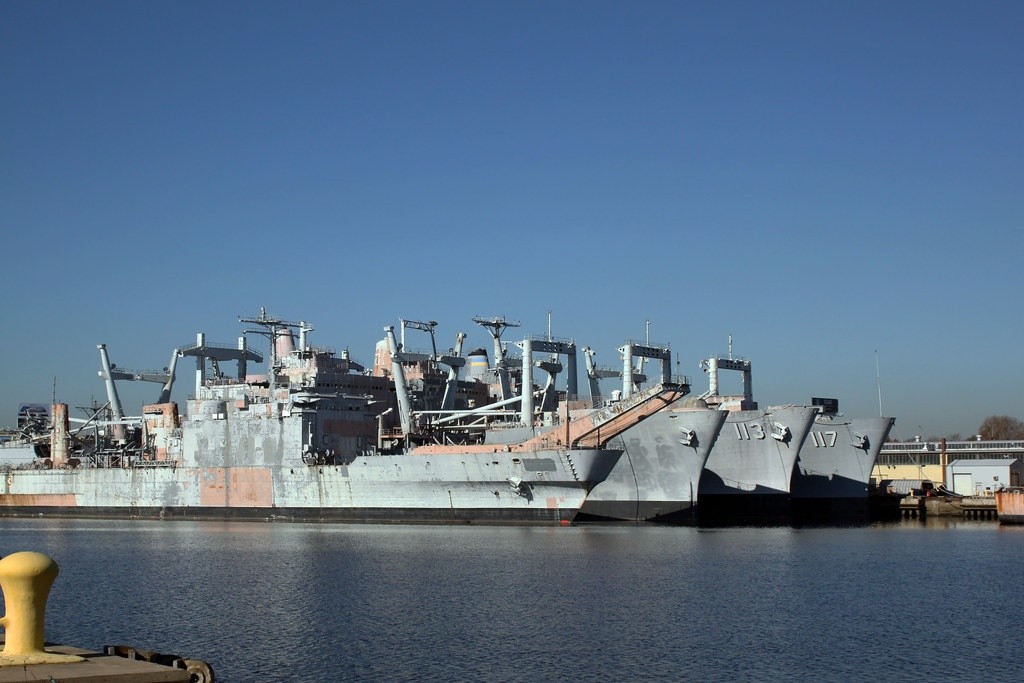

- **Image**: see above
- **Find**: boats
[374,311,898,524]
[1,306,690,526]
